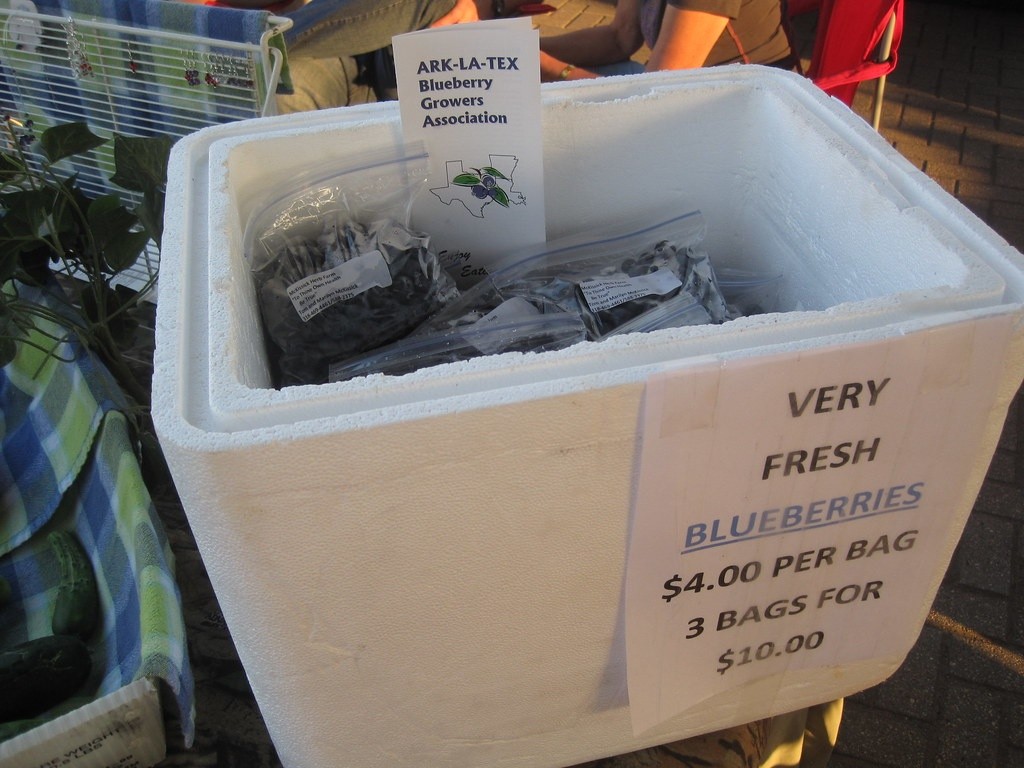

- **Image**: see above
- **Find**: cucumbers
[46,528,100,641]
[0,636,92,725]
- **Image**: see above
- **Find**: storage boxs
[147,65,1024,768]
[0,263,199,768]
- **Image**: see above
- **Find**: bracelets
[558,63,575,82]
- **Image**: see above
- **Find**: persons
[186,0,795,119]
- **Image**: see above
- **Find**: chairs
[780,0,904,133]
